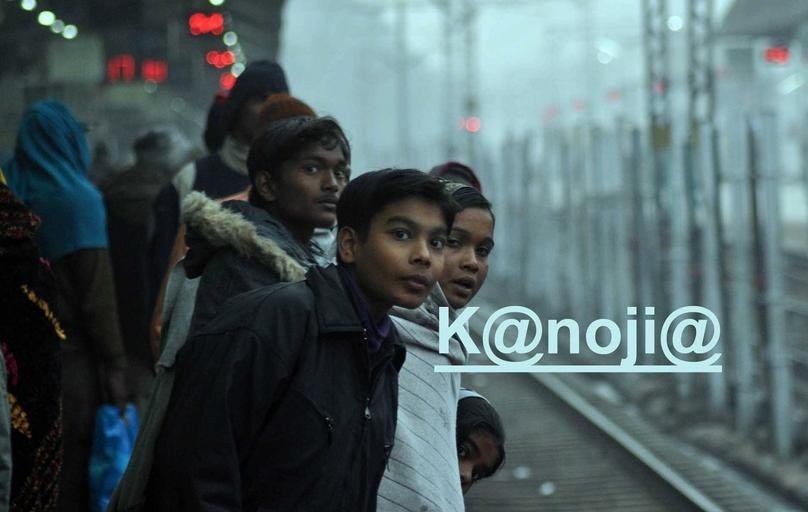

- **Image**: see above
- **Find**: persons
[1,172,92,512]
[457,388,506,498]
[181,115,351,344]
[109,169,453,512]
[99,129,180,372]
[3,101,126,404]
[147,94,319,361]
[375,174,497,512]
[201,63,292,148]
